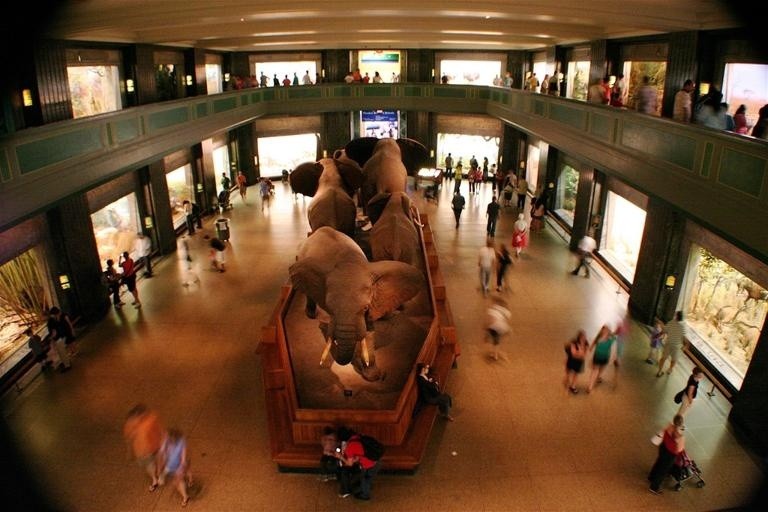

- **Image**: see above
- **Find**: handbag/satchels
[673,386,688,404]
[348,431,386,461]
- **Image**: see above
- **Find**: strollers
[669,450,706,492]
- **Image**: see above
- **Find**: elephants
[287,225,426,382]
[286,136,430,320]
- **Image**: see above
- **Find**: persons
[227,70,399,91]
[492,70,767,142]
[647,415,688,496]
[155,430,194,508]
[121,403,168,493]
[314,424,380,502]
[678,367,703,420]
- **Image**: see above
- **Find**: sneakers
[563,360,623,395]
[114,299,143,310]
[645,356,673,377]
[147,480,158,492]
[181,494,191,506]
[648,487,665,496]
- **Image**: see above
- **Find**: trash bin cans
[214,219,229,242]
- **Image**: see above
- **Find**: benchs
[260,211,462,475]
